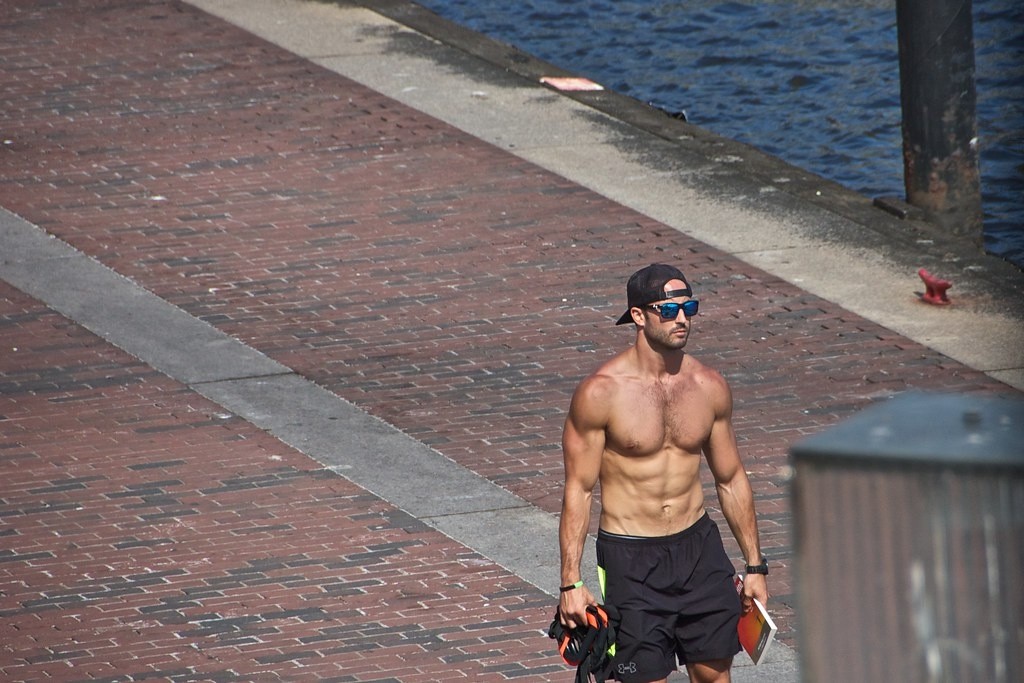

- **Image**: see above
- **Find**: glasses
[639,300,700,317]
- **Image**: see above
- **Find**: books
[734,575,778,667]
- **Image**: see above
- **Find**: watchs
[744,558,769,575]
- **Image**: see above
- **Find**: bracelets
[560,580,584,593]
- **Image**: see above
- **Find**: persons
[547,263,769,683]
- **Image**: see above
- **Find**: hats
[614,263,694,325]
